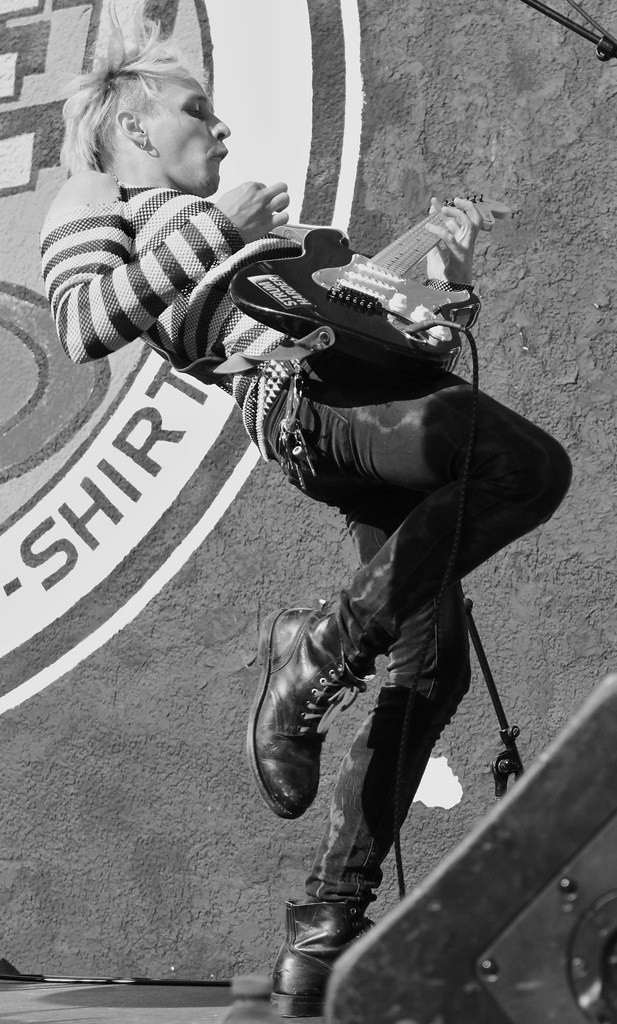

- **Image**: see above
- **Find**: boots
[271,895,379,1017]
[247,607,376,818]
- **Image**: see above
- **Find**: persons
[40,41,573,1017]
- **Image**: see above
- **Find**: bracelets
[427,278,475,294]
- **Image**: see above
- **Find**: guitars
[226,192,512,376]
